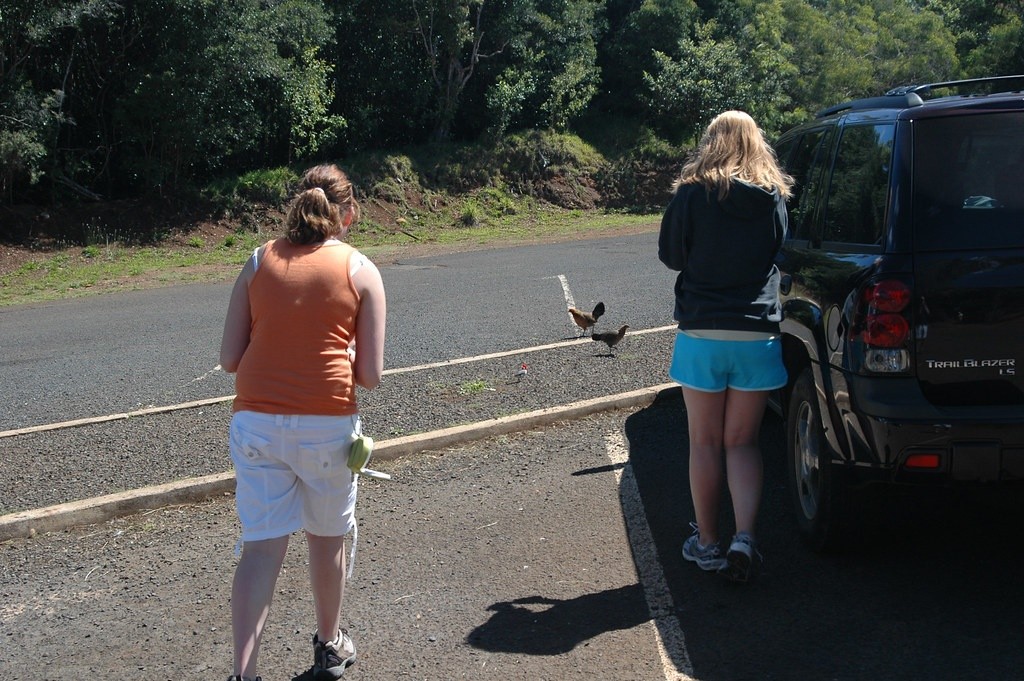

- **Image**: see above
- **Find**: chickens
[568,301,606,336]
[591,324,631,353]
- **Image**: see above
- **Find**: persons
[220,165,386,681]
[658,110,795,580]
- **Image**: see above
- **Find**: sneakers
[312,627,357,680]
[682,521,724,571]
[716,534,753,583]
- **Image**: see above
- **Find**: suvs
[760,72,1023,544]
